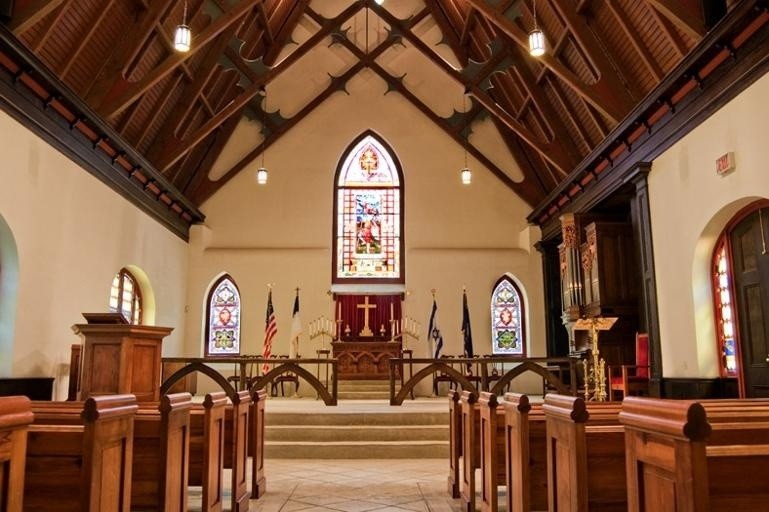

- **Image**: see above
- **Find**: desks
[0,390,268,512]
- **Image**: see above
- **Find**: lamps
[173,8,194,53]
[529,2,546,60]
[459,87,472,185]
[257,88,269,186]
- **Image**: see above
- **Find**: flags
[461,293,473,358]
[427,300,444,359]
[263,291,277,375]
[289,296,301,359]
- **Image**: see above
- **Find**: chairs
[607,331,652,402]
